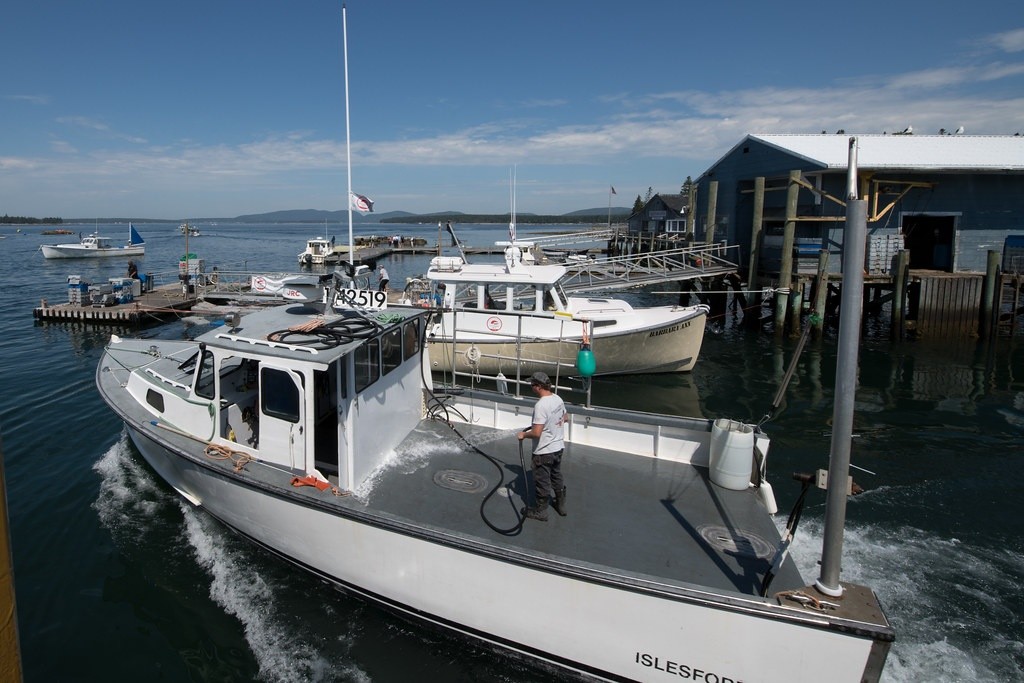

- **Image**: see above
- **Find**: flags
[350,191,375,216]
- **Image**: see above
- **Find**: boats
[298,237,335,265]
[95,201,899,683]
[39,214,146,260]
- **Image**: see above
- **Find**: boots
[552,485,568,516]
[521,496,550,521]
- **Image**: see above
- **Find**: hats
[526,372,551,387]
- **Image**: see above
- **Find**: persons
[125,261,138,279]
[388,234,404,248]
[210,267,218,285]
[377,264,390,291]
[517,372,567,521]
[371,234,375,248]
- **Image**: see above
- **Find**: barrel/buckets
[709,418,754,490]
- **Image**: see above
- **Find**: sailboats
[301,5,711,380]
